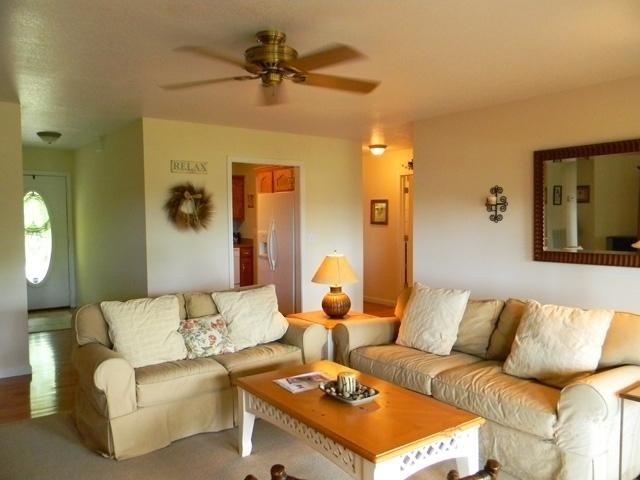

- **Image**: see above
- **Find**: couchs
[71,282,332,464]
[332,287,639,480]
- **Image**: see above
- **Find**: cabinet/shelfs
[235,236,255,287]
[231,174,246,220]
[254,165,294,194]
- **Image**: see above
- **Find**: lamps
[37,131,62,145]
[312,254,361,319]
[367,145,387,155]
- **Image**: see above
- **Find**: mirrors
[532,138,640,268]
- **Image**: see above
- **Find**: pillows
[100,291,187,372]
[169,311,232,358]
[395,281,471,360]
[211,284,292,354]
[498,298,610,391]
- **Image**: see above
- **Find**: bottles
[234,232,241,244]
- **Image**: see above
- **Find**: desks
[615,382,639,479]
[286,308,384,360]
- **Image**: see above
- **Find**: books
[271,371,334,394]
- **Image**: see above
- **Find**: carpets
[28,308,73,334]
[2,400,523,480]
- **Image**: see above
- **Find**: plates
[319,379,381,405]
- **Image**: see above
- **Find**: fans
[159,32,382,108]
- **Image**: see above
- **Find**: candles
[488,196,497,204]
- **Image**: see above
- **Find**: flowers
[161,180,216,233]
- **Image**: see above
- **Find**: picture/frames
[371,199,388,226]
[576,185,591,204]
[553,185,562,206]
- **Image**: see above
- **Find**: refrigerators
[256,192,295,316]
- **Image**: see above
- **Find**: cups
[337,372,356,394]
